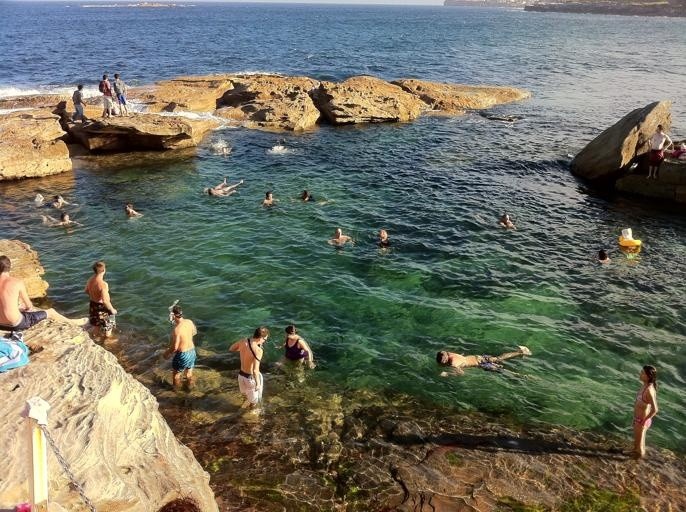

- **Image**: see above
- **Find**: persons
[622,365,658,458]
[278,325,316,370]
[499,213,516,231]
[72,85,89,124]
[645,125,672,180]
[208,178,243,195]
[168,306,198,391]
[0,256,46,330]
[623,245,639,261]
[436,345,532,378]
[335,228,388,243]
[263,190,309,203]
[596,249,610,264]
[125,203,137,215]
[228,327,270,414]
[99,75,114,118]
[113,74,130,117]
[210,139,288,154]
[37,193,69,221]
[86,262,117,337]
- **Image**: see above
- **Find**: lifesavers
[620,238,642,247]
